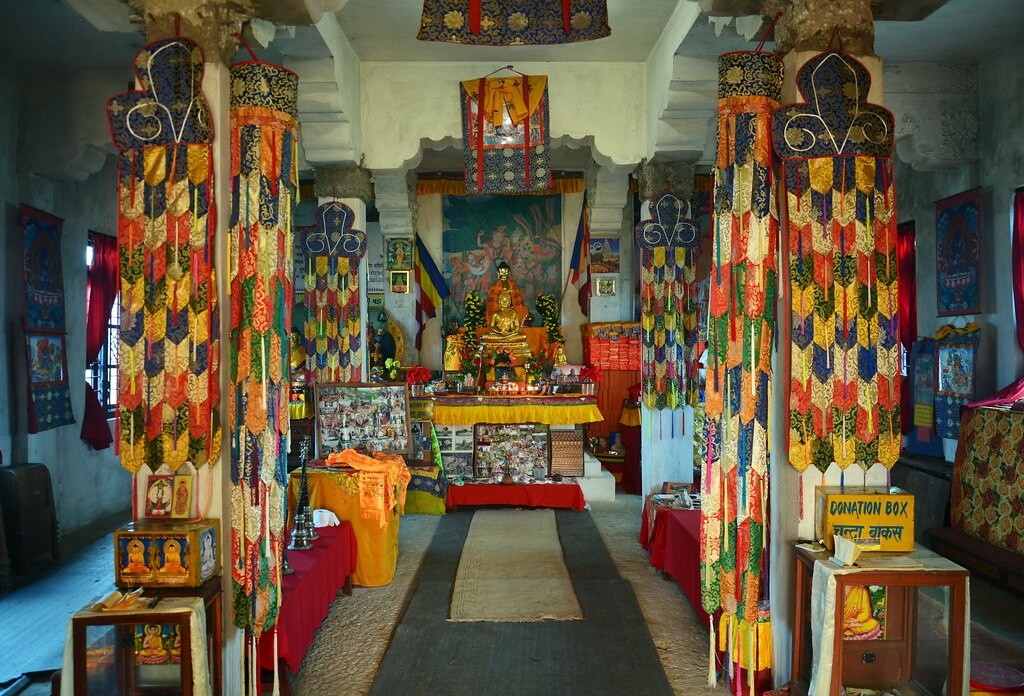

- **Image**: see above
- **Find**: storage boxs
[815,484,916,553]
[110,515,220,589]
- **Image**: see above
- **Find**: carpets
[445,482,585,512]
[445,509,585,622]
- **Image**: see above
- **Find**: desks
[639,495,718,634]
[404,465,446,516]
[790,543,974,696]
[59,576,221,696]
[248,520,359,695]
[433,395,603,481]
[287,457,410,585]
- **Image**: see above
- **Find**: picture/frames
[383,235,414,273]
[313,382,413,457]
[389,271,410,295]
[594,277,617,297]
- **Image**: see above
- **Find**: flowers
[503,452,511,476]
[454,343,483,386]
[384,359,402,381]
[528,351,554,378]
[405,365,433,385]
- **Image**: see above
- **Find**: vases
[411,380,426,398]
[501,475,514,484]
[457,383,476,394]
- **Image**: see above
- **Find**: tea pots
[489,372,517,396]
